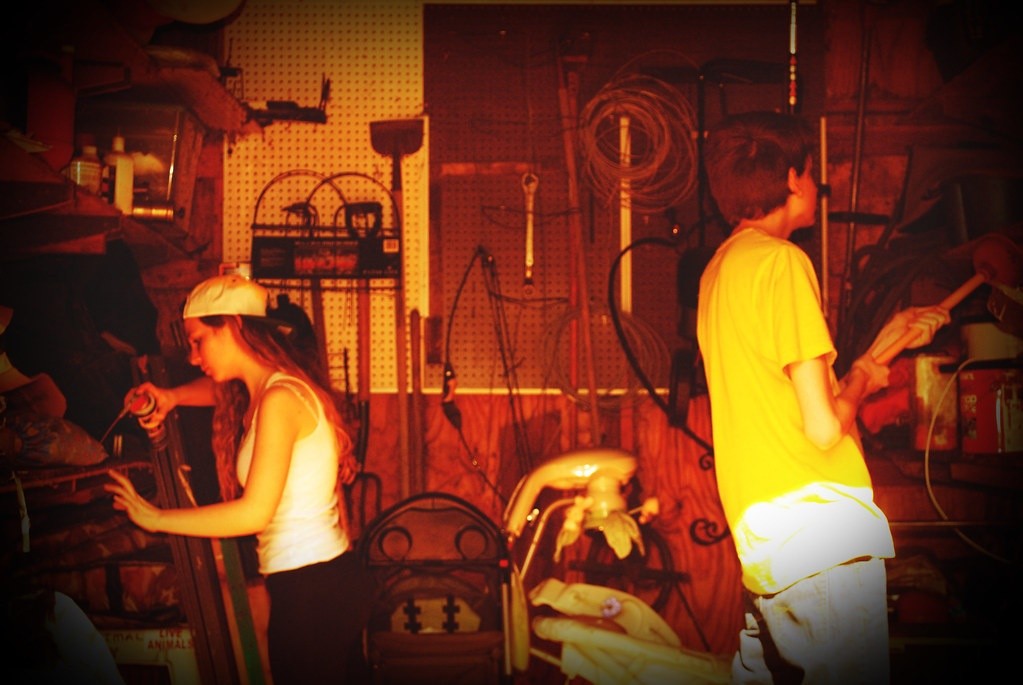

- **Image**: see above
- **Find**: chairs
[357,492,513,685]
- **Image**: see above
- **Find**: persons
[106,275,367,685]
[696,109,951,685]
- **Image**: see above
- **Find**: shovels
[874,237,1023,366]
[368,117,426,499]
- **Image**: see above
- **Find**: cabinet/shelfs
[890,519,1022,648]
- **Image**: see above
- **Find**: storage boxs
[76,100,209,240]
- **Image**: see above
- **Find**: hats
[183,273,296,339]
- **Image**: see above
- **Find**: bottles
[70,146,103,195]
[97,136,133,217]
[129,391,167,450]
